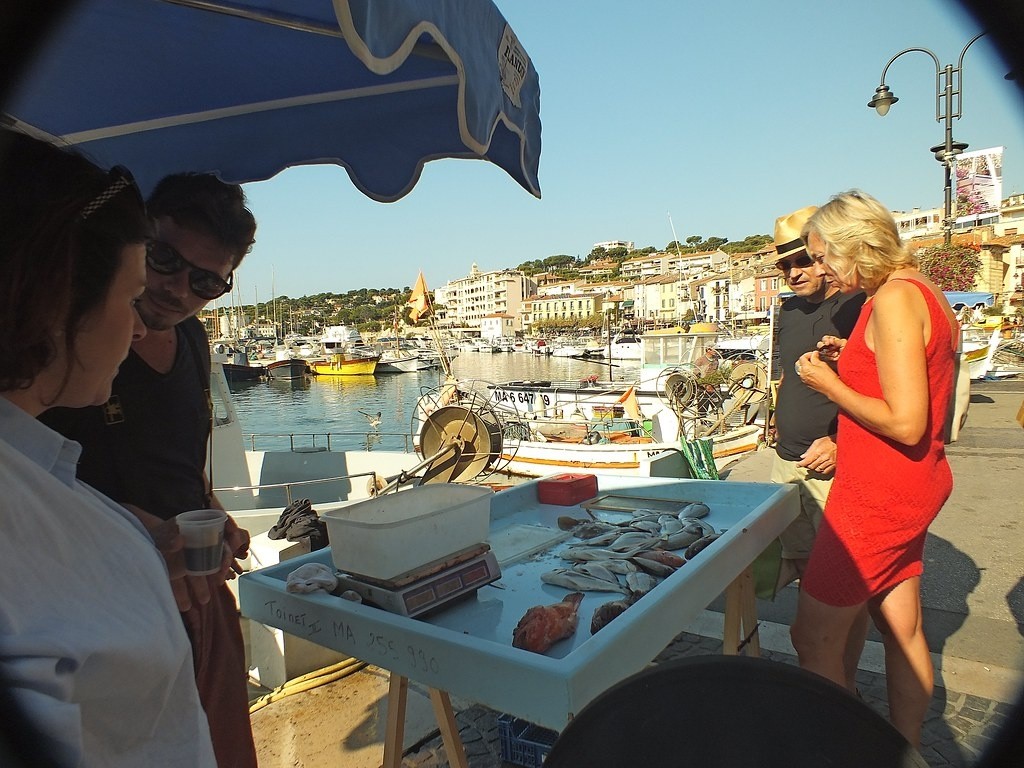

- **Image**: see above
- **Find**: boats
[410,358,770,481]
[208,267,1024,419]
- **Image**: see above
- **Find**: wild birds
[358,410,383,430]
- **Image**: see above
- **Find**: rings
[818,466,823,471]
[795,365,800,376]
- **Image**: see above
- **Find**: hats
[773,207,818,262]
[705,341,720,349]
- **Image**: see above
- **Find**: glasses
[144,232,235,301]
[775,255,812,271]
[67,164,143,229]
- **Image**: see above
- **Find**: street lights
[866,27,1024,245]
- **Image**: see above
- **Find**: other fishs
[511,498,728,656]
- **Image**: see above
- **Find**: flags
[409,273,428,325]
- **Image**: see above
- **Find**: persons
[35,172,258,768]
[790,191,961,750]
[771,205,869,698]
[0,127,217,768]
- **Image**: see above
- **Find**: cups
[175,509,228,575]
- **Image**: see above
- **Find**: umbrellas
[942,291,994,311]
[5,0,542,204]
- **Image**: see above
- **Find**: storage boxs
[318,483,494,580]
[497,713,559,768]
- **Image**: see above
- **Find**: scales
[334,543,502,619]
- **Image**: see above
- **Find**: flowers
[916,243,981,292]
